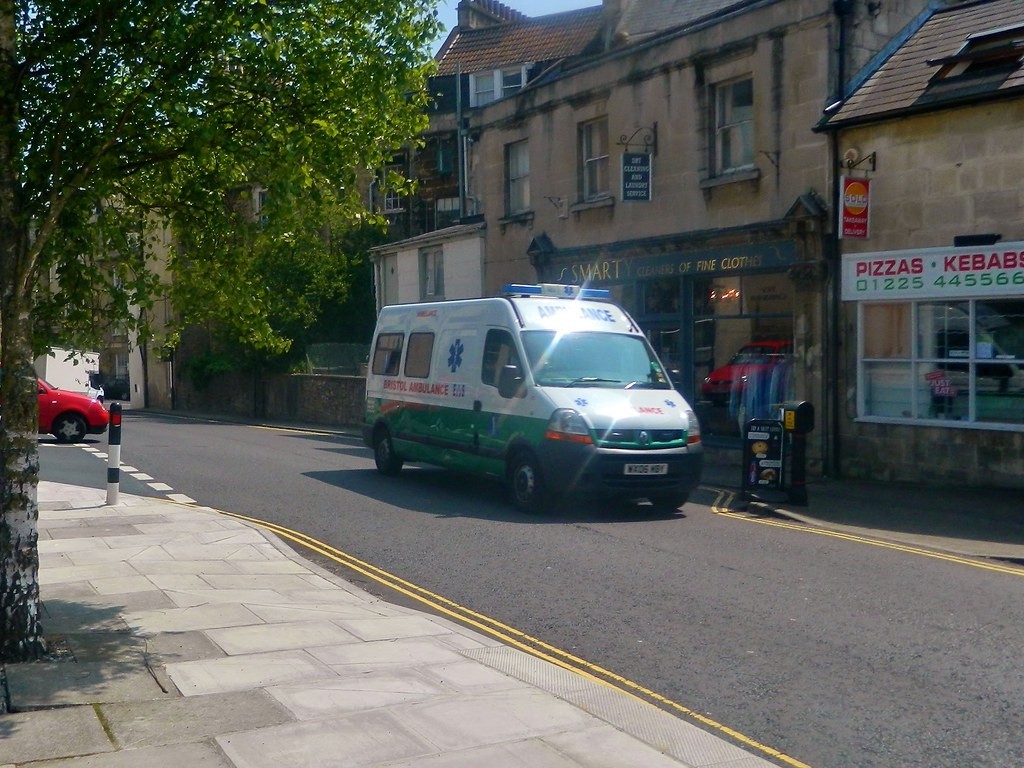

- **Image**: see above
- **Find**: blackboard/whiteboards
[621,151,653,202]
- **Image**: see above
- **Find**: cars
[918,305,1024,392]
[0,360,111,444]
[950,302,1024,370]
[702,340,794,407]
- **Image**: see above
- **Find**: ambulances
[362,283,703,515]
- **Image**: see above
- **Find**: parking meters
[784,400,815,507]
